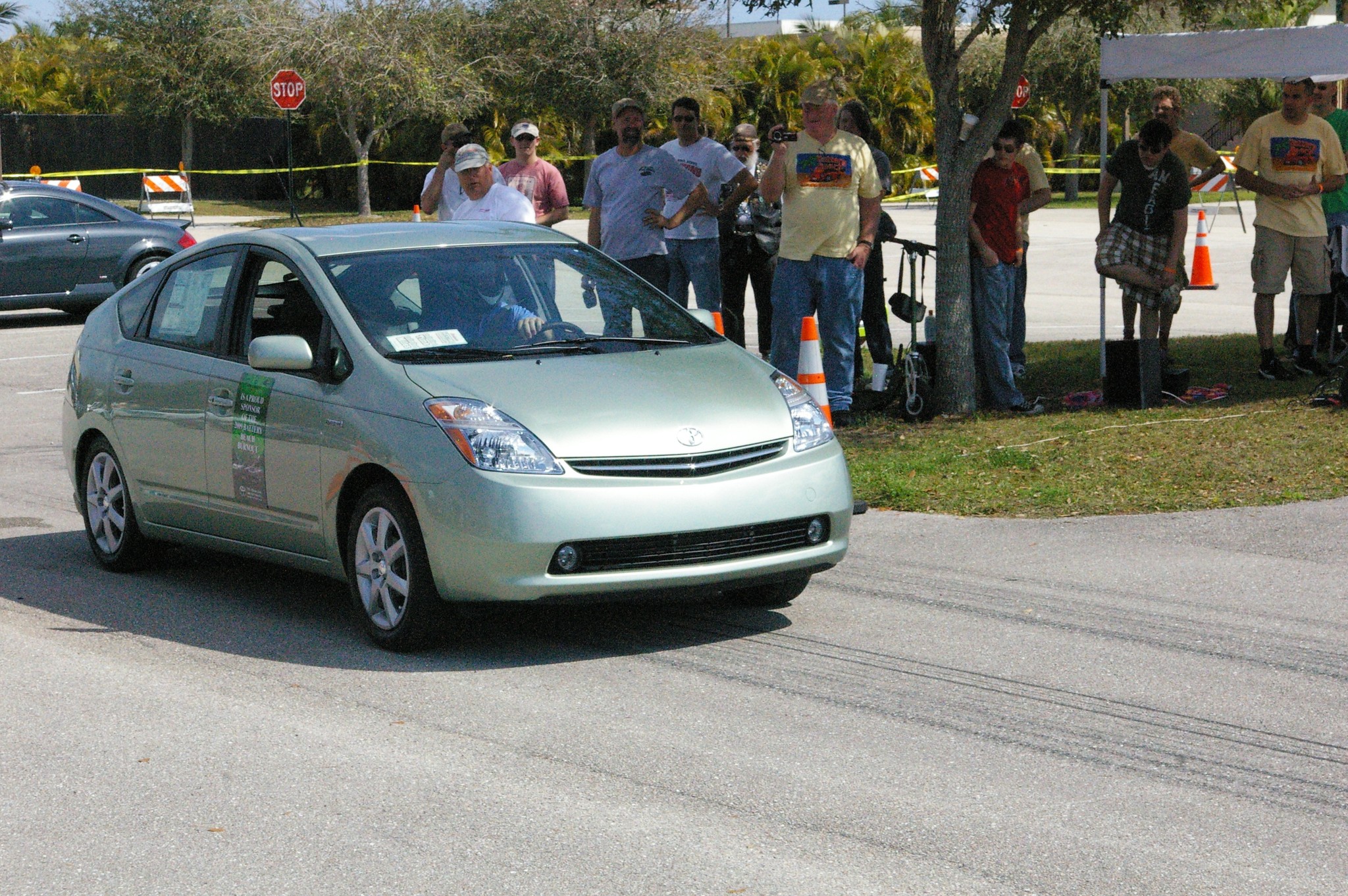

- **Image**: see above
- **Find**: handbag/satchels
[889,292,926,324]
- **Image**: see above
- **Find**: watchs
[857,240,875,251]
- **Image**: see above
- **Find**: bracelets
[1318,183,1323,194]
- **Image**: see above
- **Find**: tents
[1099,20,1348,382]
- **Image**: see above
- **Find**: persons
[982,140,1051,390]
[1304,81,1348,359]
[1093,118,1192,364]
[967,120,1044,413]
[757,84,881,427]
[1125,86,1227,361]
[427,260,557,354]
[421,84,899,425]
[1232,77,1348,380]
[577,97,708,339]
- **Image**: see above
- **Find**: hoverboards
[880,231,940,423]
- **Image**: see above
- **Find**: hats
[733,123,757,142]
[454,143,488,173]
[441,121,470,143]
[511,122,539,138]
[612,98,642,118]
[801,81,837,106]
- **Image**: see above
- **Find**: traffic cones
[1185,208,1220,291]
[795,315,840,437]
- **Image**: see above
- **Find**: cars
[0,176,199,323]
[64,217,855,659]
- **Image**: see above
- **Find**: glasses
[1314,83,1327,90]
[992,142,1014,154]
[1154,106,1175,112]
[732,145,750,152]
[1137,140,1163,154]
[673,114,694,123]
[515,134,534,142]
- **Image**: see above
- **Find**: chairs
[264,287,318,337]
[1286,208,1348,366]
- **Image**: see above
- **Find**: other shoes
[1258,353,1284,380]
[1004,400,1043,415]
[1011,360,1027,380]
[1158,349,1175,364]
[830,409,851,427]
[1294,352,1322,376]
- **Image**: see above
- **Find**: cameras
[453,136,471,148]
[772,128,797,142]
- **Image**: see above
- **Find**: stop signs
[271,69,307,110]
[1011,74,1031,110]
